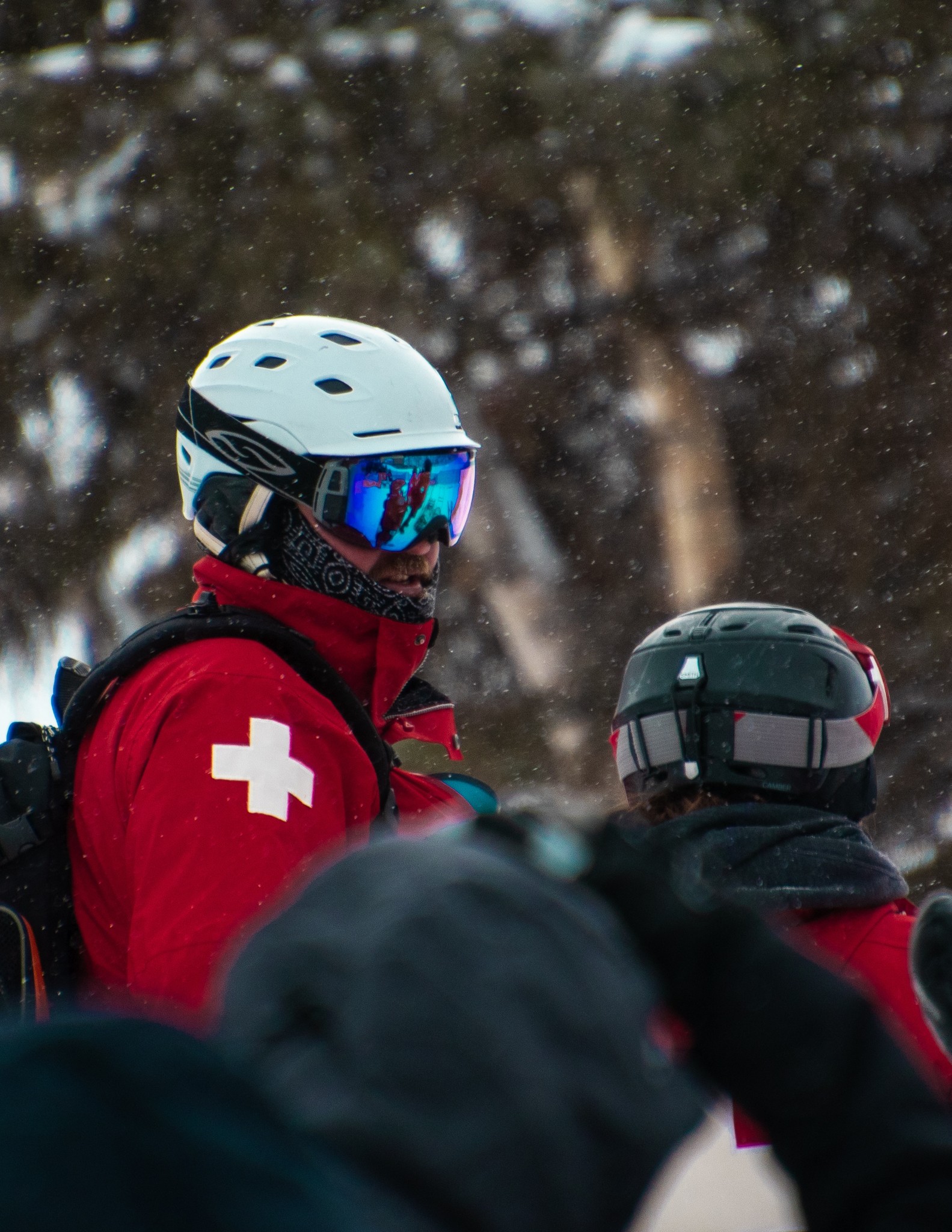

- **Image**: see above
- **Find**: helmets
[176,312,481,579]
[610,601,890,823]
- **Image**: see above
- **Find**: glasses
[175,382,481,552]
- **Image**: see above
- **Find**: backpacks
[0,594,403,1046]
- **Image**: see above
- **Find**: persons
[19,303,490,1036]
[374,480,405,550]
[400,459,436,533]
[604,594,951,1232]
